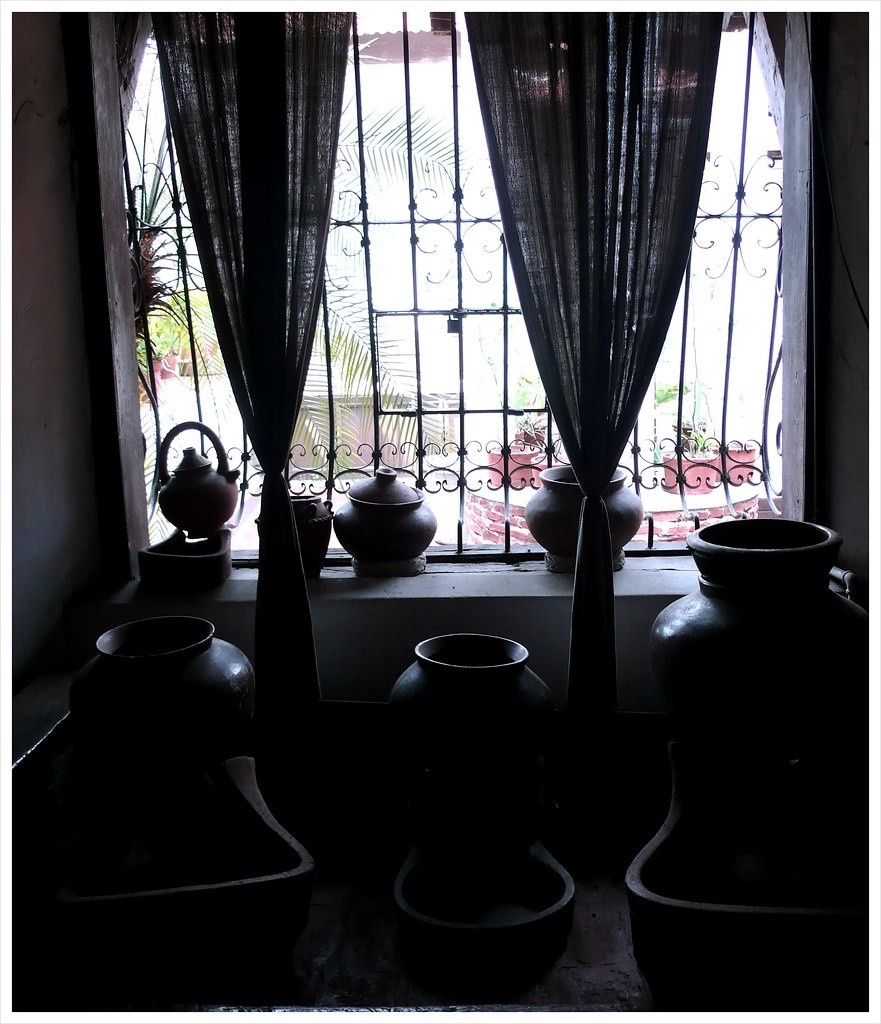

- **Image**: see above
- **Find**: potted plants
[483,375,570,491]
[134,278,211,409]
[660,415,757,497]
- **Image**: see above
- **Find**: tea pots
[154,422,243,539]
